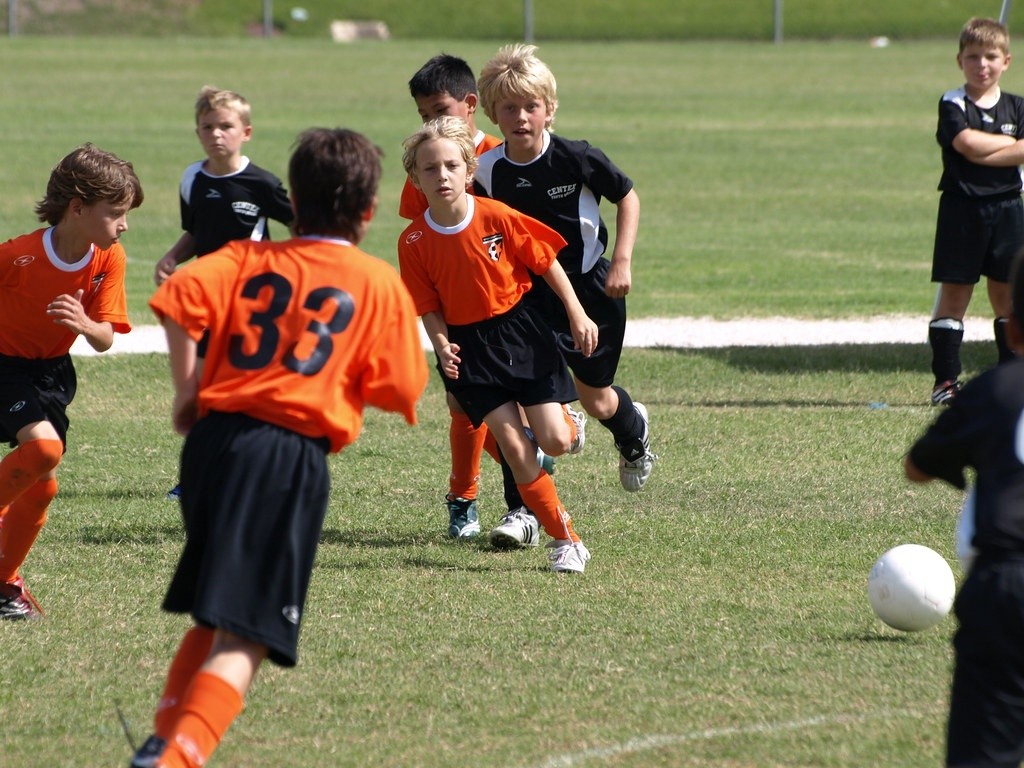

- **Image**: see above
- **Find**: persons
[0,142,144,620]
[470,43,648,546]
[904,248,1024,768]
[399,54,556,539]
[929,17,1024,406]
[157,92,296,376]
[130,128,427,768]
[399,117,598,571]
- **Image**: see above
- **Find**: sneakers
[0,576,46,625]
[563,404,588,454]
[443,493,480,540]
[489,506,540,550]
[931,380,961,406]
[545,537,592,573]
[615,402,658,493]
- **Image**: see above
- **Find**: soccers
[867,544,955,632]
